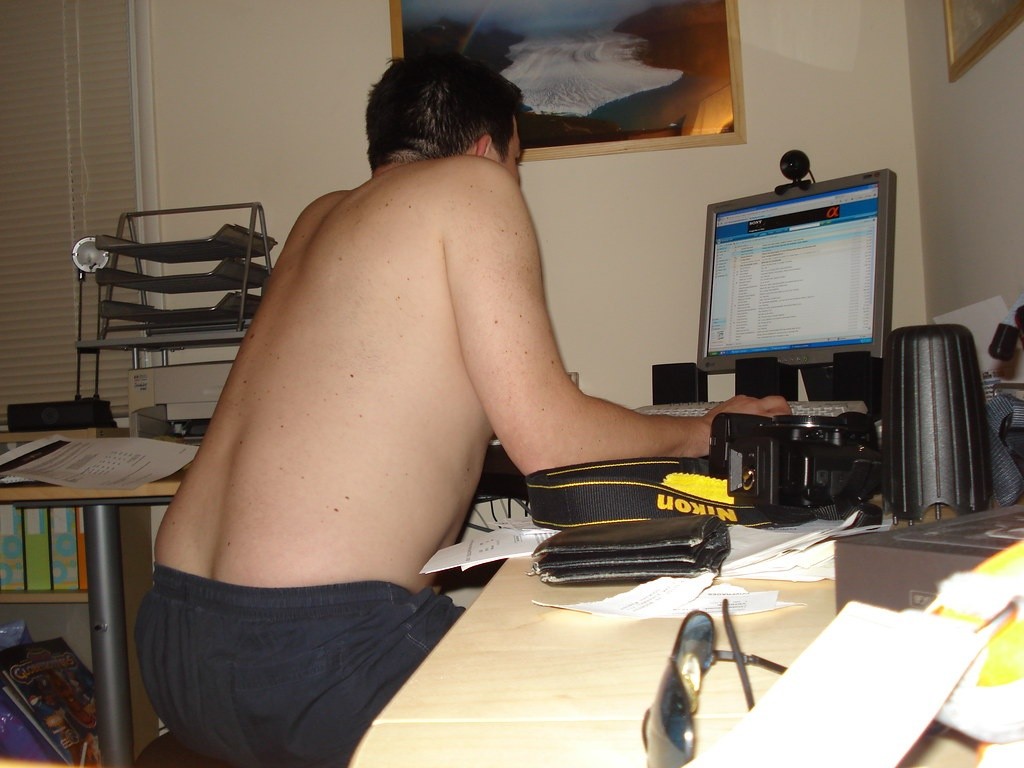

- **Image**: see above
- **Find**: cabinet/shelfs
[0,426,160,765]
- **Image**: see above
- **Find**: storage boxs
[833,503,1024,610]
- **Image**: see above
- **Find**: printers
[128,360,235,446]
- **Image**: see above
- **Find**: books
[0,636,102,768]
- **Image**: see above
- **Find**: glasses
[644,598,786,768]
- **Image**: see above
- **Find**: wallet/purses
[526,515,732,586]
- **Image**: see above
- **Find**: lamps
[6,236,118,427]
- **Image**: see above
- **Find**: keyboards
[634,401,869,418]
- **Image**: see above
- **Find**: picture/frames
[389,0,747,162]
[943,0,1024,84]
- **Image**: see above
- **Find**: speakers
[735,356,798,402]
[652,361,708,405]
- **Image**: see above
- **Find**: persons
[130,54,793,768]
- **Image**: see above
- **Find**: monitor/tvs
[698,168,897,402]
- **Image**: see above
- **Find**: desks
[0,480,183,768]
[351,507,1024,768]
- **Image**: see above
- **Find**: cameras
[709,412,883,525]
